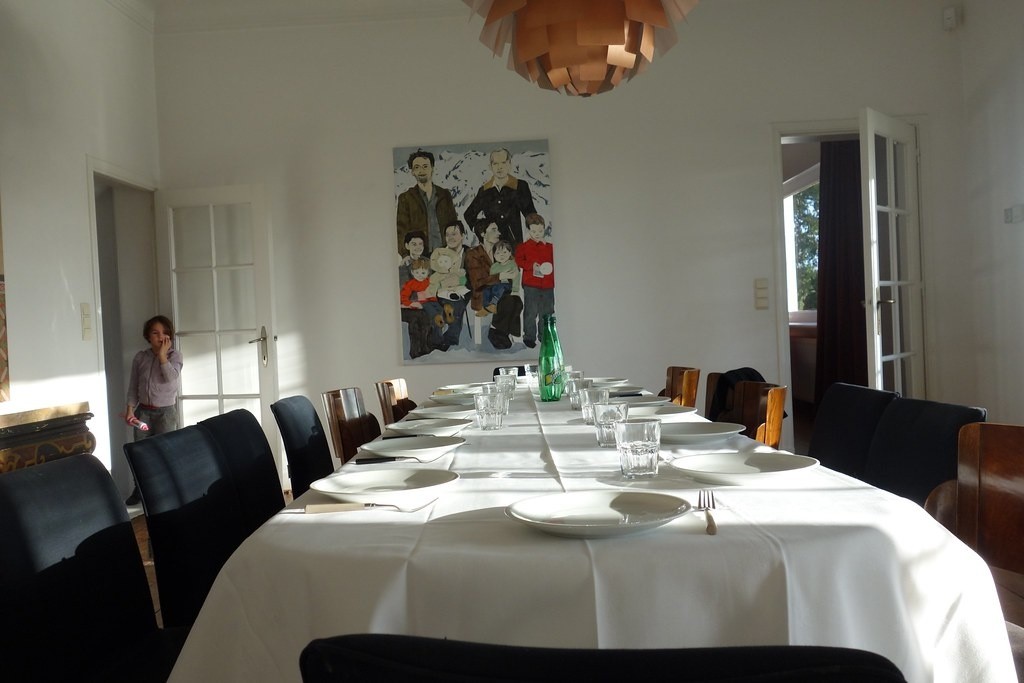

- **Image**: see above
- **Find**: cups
[592,402,628,448]
[613,419,661,480]
[476,366,518,429]
[524,363,609,424]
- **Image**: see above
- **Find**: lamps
[462,0,698,99]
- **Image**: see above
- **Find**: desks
[165,381,1021,682]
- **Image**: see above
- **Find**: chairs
[493,365,539,381]
[0,378,417,683]
[664,365,1023,683]
[300,632,906,682]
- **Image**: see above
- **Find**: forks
[697,490,717,535]
[356,451,450,463]
[305,498,438,512]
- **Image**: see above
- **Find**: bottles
[539,316,566,402]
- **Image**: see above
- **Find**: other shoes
[126,487,140,505]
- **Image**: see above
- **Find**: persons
[125,316,183,506]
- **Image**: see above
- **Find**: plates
[585,376,672,405]
[385,419,475,436]
[504,491,691,536]
[670,452,822,486]
[309,469,460,503]
[627,406,698,419]
[428,383,485,405]
[360,436,466,461]
[408,405,477,419]
[659,422,746,445]
